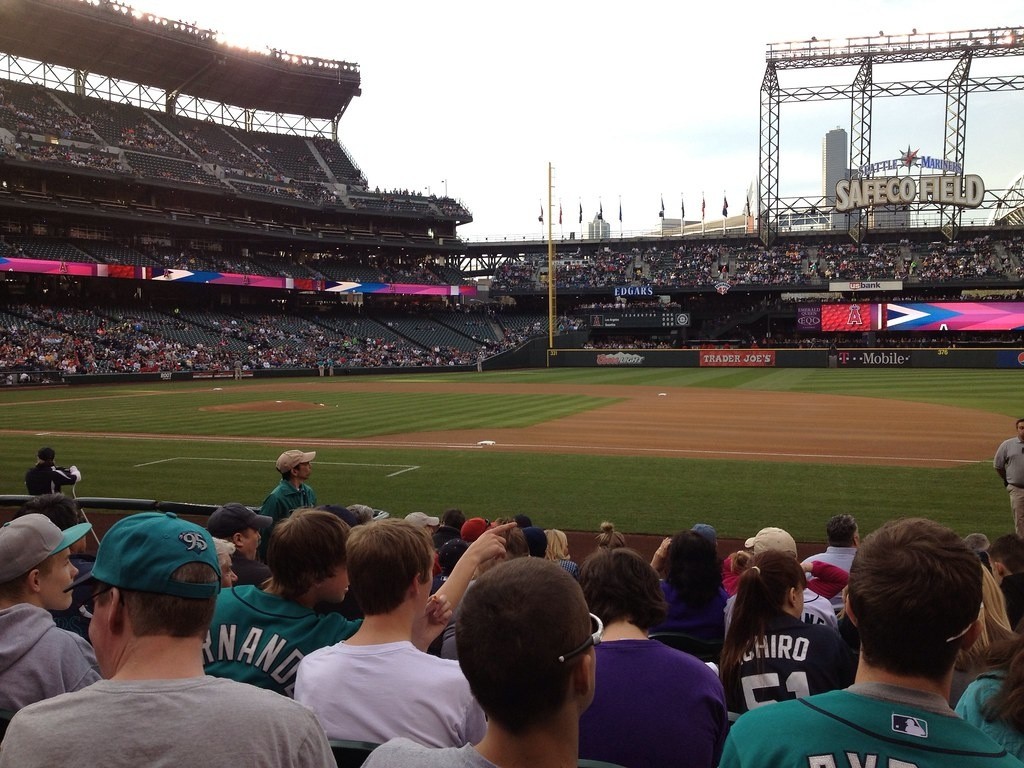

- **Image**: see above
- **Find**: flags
[539,197,751,225]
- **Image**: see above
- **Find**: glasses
[1022,447,1024,454]
[78,587,124,618]
[556,611,603,663]
[484,517,491,530]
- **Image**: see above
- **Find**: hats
[405,512,440,527]
[208,502,274,539]
[691,523,716,544]
[0,513,92,585]
[512,514,532,528]
[316,503,358,527]
[442,509,465,528]
[275,450,316,474]
[745,526,797,558]
[438,538,470,568]
[523,527,547,558]
[461,517,491,542]
[38,447,55,461]
[62,512,222,598]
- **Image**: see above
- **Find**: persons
[0,419,1023,767]
[0,84,550,386]
[551,235,1024,349]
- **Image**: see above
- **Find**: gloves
[70,465,81,482]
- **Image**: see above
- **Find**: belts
[1011,482,1024,488]
